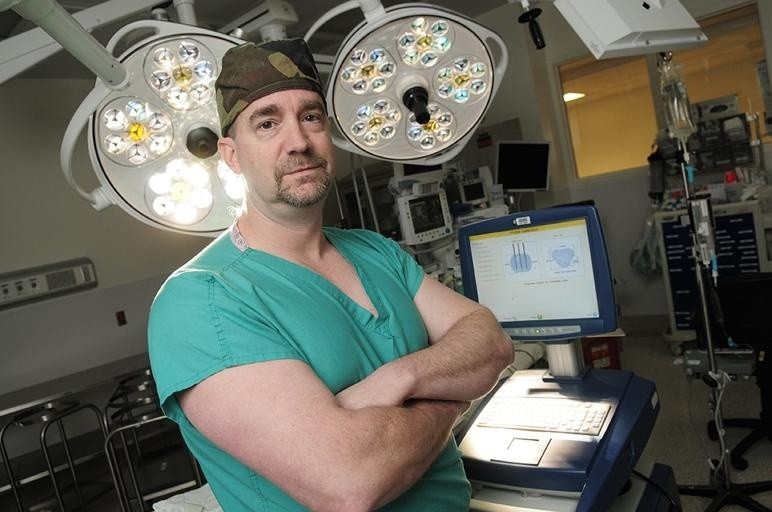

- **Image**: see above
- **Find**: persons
[147,38,515,512]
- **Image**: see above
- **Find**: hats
[215,37,327,137]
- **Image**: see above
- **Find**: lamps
[218,0,509,166]
[0,1,250,238]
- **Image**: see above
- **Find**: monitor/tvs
[495,140,551,193]
[397,189,454,246]
[458,205,621,343]
[393,161,445,186]
[552,0,709,63]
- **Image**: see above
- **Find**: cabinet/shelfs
[0,351,178,493]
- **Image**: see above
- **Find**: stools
[697,273,771,470]
